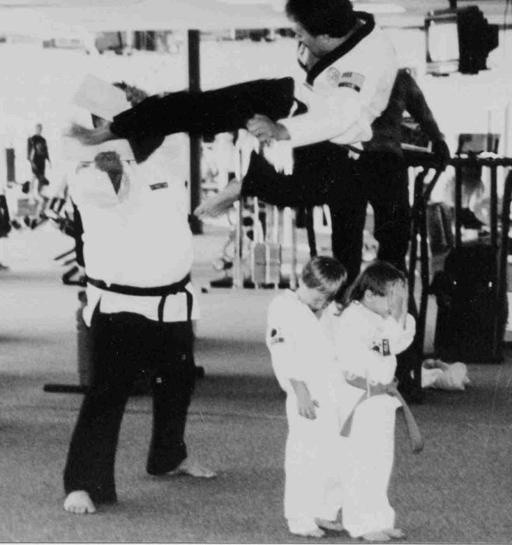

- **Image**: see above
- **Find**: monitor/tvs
[424,13,499,74]
